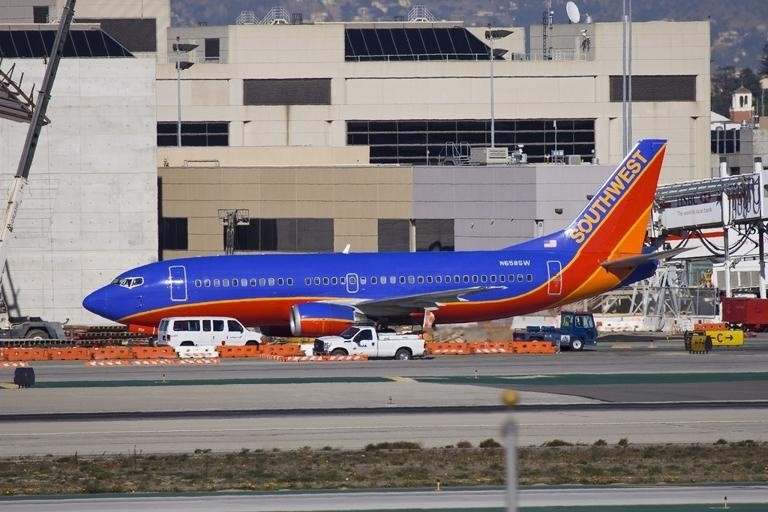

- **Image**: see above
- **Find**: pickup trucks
[312,322,427,360]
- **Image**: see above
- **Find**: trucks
[513,308,594,353]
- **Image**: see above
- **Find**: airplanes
[83,138,699,343]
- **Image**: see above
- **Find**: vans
[156,313,264,351]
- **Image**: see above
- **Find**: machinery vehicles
[0,0,76,339]
